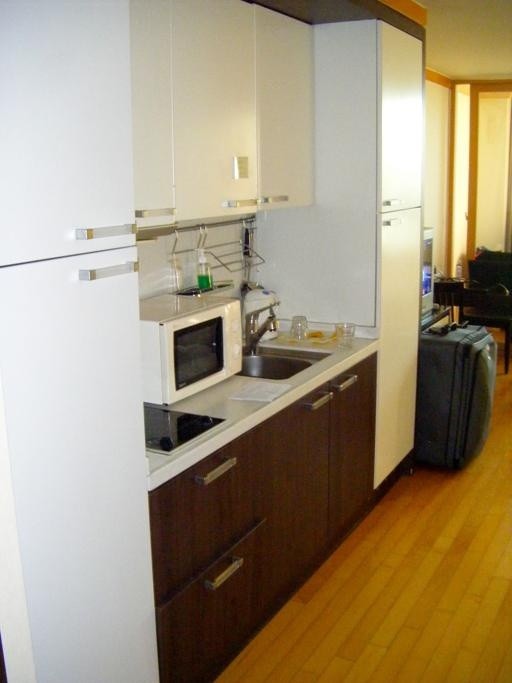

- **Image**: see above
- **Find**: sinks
[235,345,330,380]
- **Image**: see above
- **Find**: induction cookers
[143,402,225,453]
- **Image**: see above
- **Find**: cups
[334,322,355,354]
[290,315,311,342]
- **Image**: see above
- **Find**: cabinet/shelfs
[131,0,177,231]
[174,0,313,224]
[0,0,161,680]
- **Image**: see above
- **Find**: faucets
[245,302,280,352]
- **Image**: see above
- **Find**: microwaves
[140,293,243,407]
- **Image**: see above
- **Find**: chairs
[461,250,511,374]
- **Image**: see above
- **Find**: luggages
[415,321,498,473]
